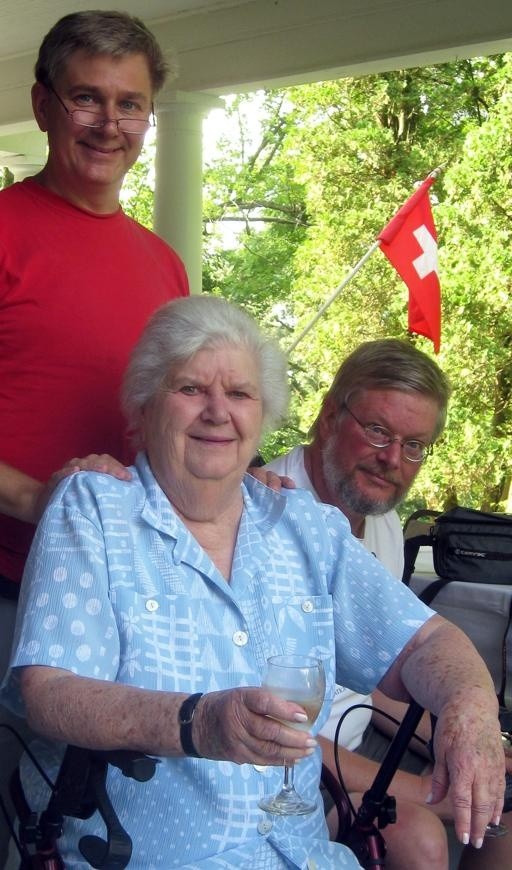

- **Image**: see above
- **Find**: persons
[0,9,188,870]
[1,296,507,869]
[248,338,512,868]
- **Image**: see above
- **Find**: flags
[376,176,441,354]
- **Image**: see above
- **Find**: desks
[404,572,512,714]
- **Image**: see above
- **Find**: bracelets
[177,692,203,760]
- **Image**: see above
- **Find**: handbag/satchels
[432,506,512,585]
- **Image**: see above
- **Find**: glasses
[46,79,156,135]
[342,402,434,464]
